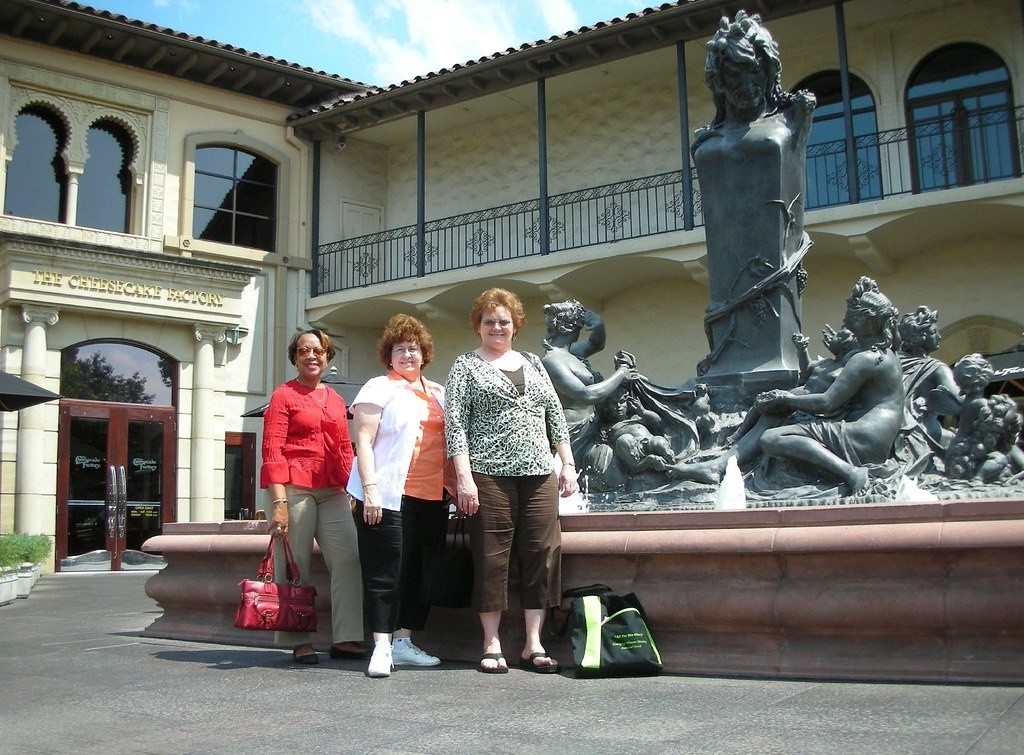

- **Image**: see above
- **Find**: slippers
[518,651,561,673]
[476,653,509,674]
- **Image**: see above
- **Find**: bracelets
[562,461,575,466]
[361,482,376,488]
[274,499,288,504]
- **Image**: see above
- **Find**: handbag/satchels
[234,531,318,634]
[546,583,664,679]
[440,516,474,597]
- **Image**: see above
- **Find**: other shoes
[330,645,373,661]
[293,648,319,665]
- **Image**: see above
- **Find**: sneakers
[368,648,394,677]
[390,641,440,666]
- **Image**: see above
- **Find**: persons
[543,11,1023,492]
[347,315,464,677]
[260,327,371,664]
[444,288,579,675]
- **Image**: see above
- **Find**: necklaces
[294,377,326,400]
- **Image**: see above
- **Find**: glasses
[295,348,326,356]
[482,318,514,327]
[390,346,423,354]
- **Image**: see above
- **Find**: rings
[277,527,282,533]
[367,513,371,516]
